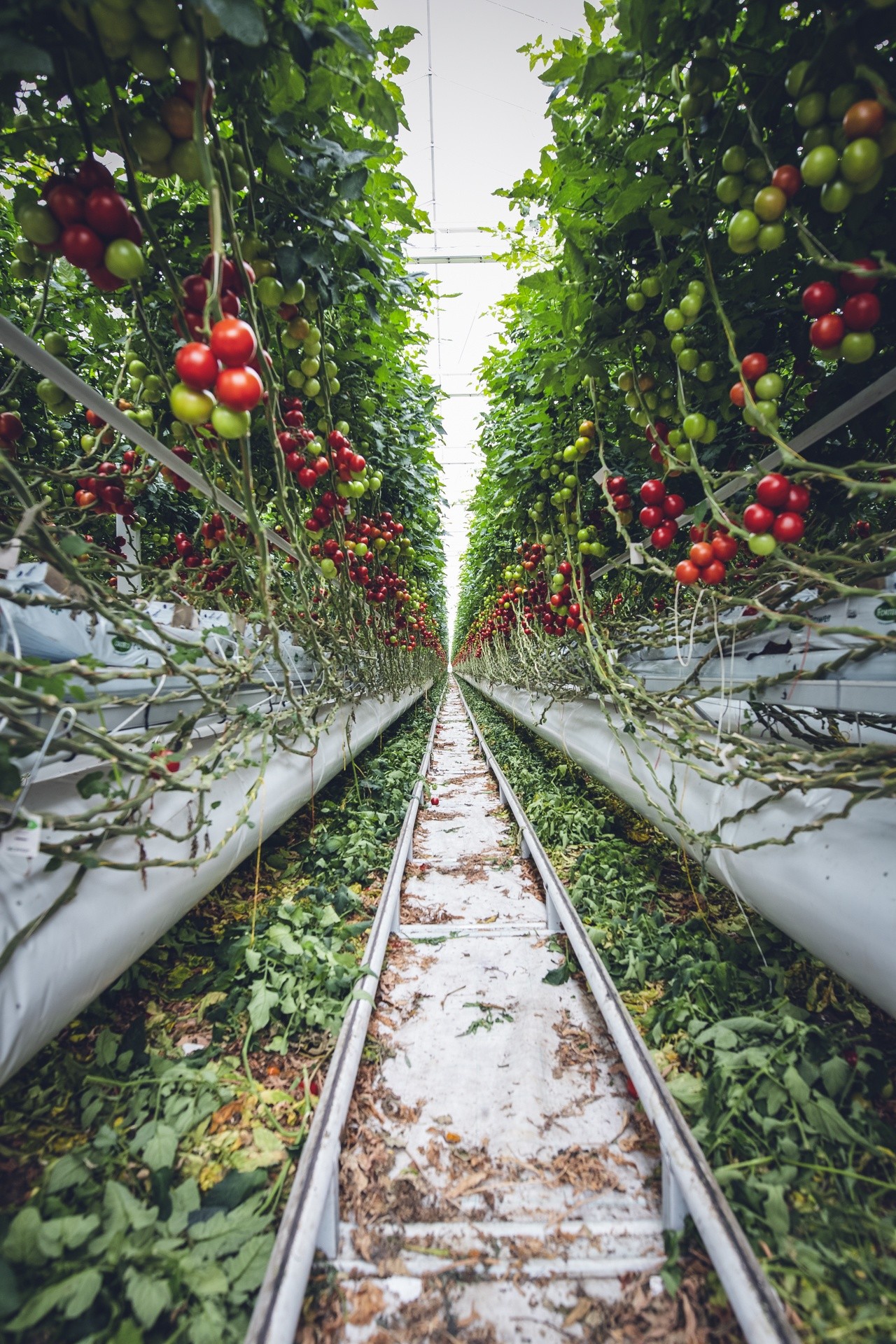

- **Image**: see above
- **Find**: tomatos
[0,0,896,776]
